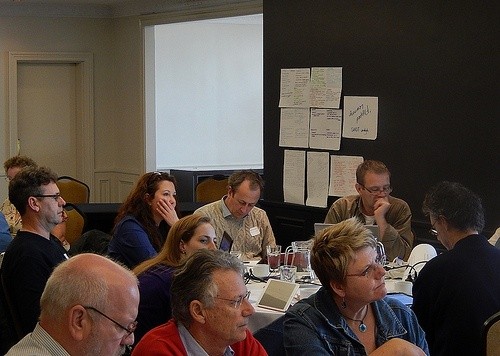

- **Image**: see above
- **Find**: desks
[245,264,415,333]
[75,195,204,216]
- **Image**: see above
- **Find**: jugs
[374,238,386,266]
[284,239,316,282]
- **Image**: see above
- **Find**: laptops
[218,231,233,253]
[314,223,380,243]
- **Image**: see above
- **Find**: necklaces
[341,304,370,333]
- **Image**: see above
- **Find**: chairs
[61,203,85,244]
[479,312,500,356]
[194,174,230,204]
[57,176,90,206]
[406,244,437,275]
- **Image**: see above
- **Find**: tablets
[257,278,300,312]
[385,291,413,306]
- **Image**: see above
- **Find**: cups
[266,245,281,274]
[280,265,297,283]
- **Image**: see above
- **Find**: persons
[105,170,221,356]
[279,219,431,356]
[0,156,73,355]
[410,183,500,356]
[325,158,415,262]
[2,253,140,356]
[133,249,269,356]
[191,169,279,260]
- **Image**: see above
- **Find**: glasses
[4,178,14,183]
[84,307,138,338]
[212,291,251,309]
[428,217,440,236]
[358,182,393,196]
[342,254,386,280]
[26,193,61,201]
